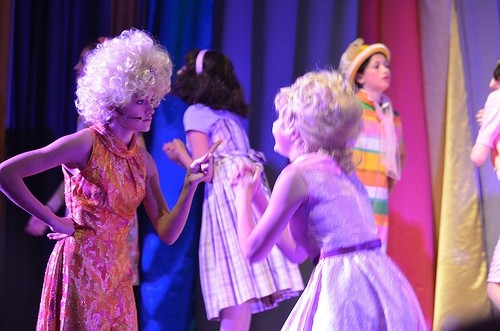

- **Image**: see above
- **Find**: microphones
[125,116,142,119]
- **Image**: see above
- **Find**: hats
[337,38,390,89]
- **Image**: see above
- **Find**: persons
[337,38,407,254]
[469,58,500,314]
[230,69,427,331]
[160,49,303,331]
[1,28,222,331]
[24,35,113,236]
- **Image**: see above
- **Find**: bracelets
[45,204,57,213]
